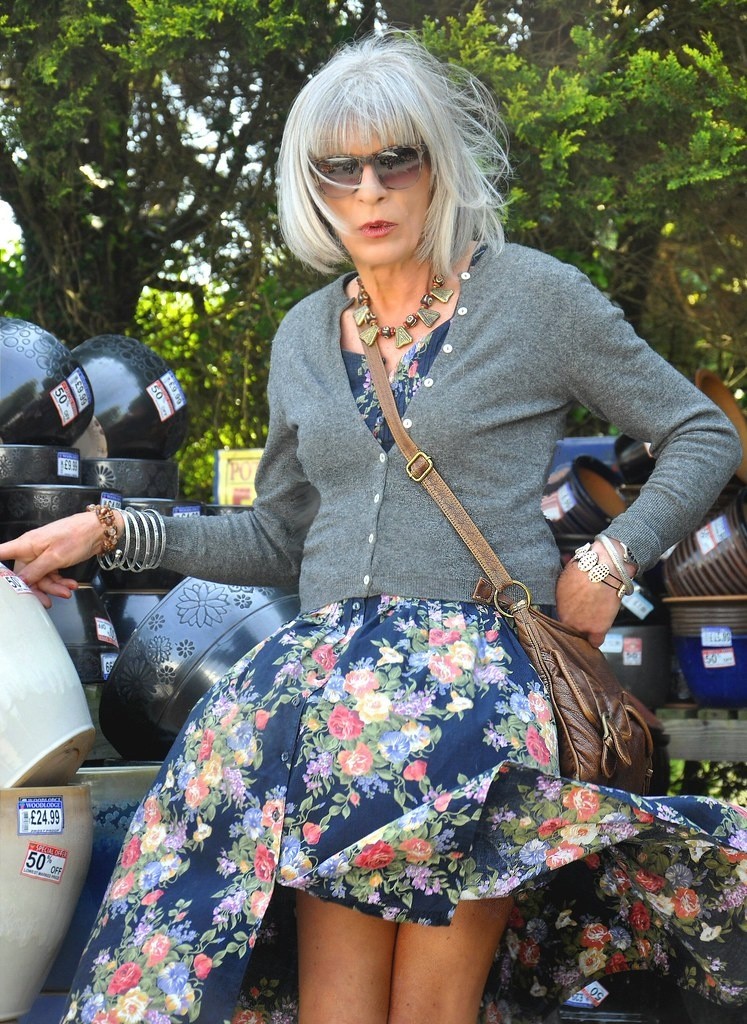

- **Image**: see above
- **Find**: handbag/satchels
[517,603,655,794]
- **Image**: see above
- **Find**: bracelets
[593,533,635,596]
[82,503,120,558]
[560,542,629,598]
[97,507,168,573]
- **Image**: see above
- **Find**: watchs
[620,539,642,569]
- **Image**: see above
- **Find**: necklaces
[351,265,455,347]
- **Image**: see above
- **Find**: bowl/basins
[0,312,747,1024]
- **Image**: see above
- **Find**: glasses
[311,145,430,197]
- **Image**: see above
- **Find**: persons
[0,33,737,1022]
